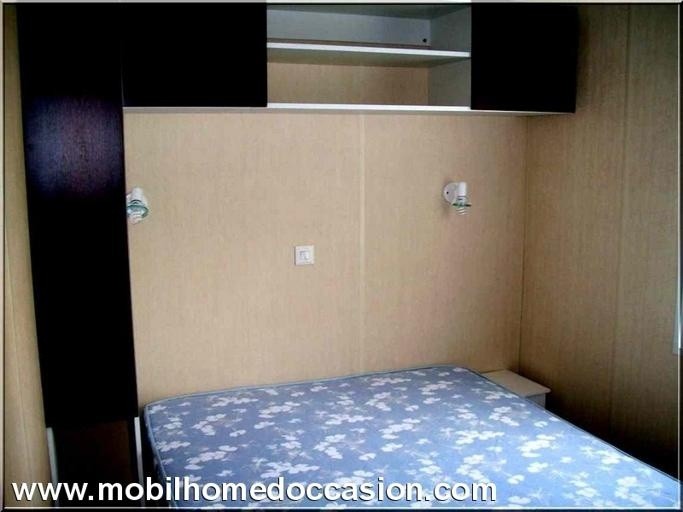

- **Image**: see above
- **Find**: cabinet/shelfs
[266,2,472,112]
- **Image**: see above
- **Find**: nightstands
[478,368,550,412]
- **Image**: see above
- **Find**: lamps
[127,187,148,227]
[444,182,472,216]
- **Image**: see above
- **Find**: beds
[140,364,681,509]
